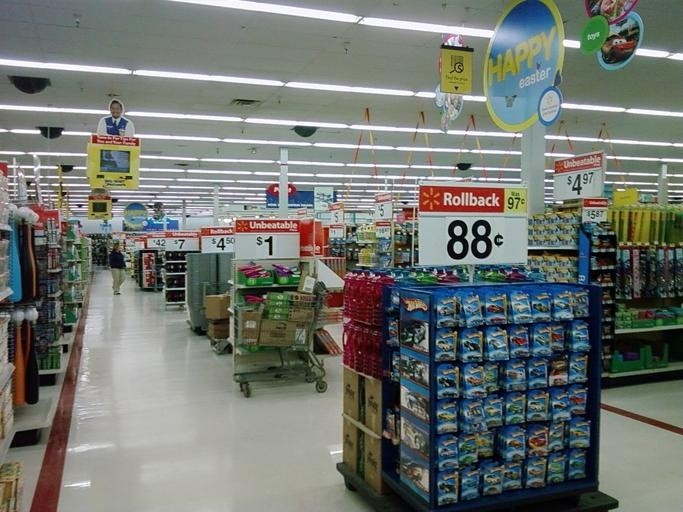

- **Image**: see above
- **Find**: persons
[110,242,127,295]
[97,98,135,138]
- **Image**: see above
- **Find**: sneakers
[112,292,122,296]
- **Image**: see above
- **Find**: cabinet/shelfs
[36,241,67,375]
[336,282,618,512]
[61,254,85,326]
[609,244,683,379]
[0,220,18,468]
[142,253,163,292]
[354,240,379,268]
[165,259,186,303]
[580,222,614,378]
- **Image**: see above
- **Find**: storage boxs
[203,294,230,320]
[258,319,298,346]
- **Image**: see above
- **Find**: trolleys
[232,283,329,397]
[203,280,231,353]
